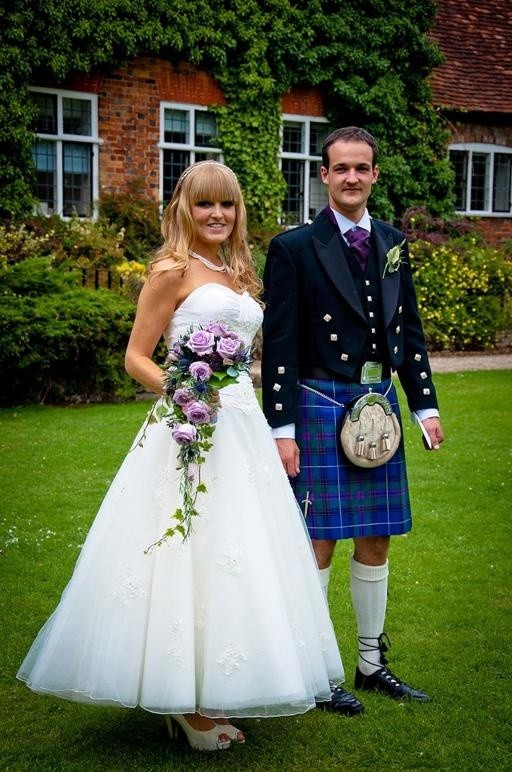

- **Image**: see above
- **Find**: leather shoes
[355,664,433,705]
[314,677,366,717]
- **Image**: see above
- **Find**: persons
[262,126,445,719]
[15,159,347,755]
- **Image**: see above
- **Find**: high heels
[164,711,250,751]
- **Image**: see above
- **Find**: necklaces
[188,247,227,273]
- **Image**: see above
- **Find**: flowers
[118,318,248,556]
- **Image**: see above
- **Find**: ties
[345,226,372,273]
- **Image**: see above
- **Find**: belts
[298,359,391,385]
